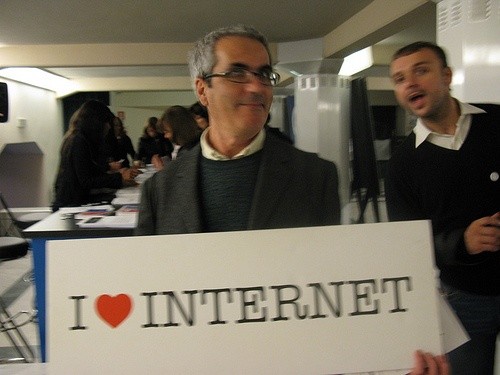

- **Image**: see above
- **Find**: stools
[0,212,50,364]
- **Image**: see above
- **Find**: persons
[385,42,500,374]
[51,99,209,213]
[136,26,450,374]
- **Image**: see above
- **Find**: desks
[21,163,163,362]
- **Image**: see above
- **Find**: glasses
[203,67,281,88]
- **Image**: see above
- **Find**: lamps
[1,65,72,93]
